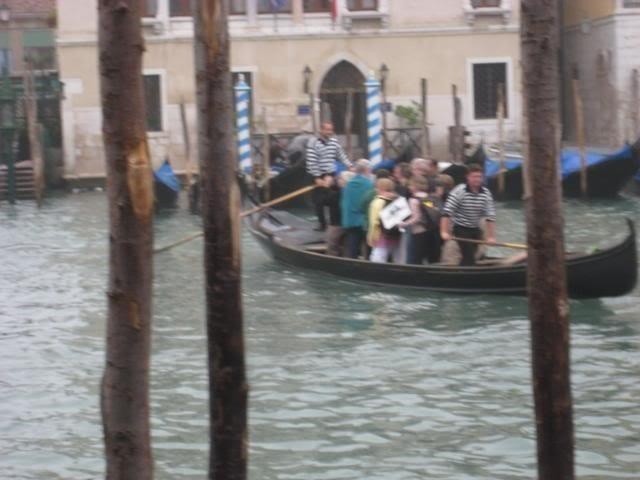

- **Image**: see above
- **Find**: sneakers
[312,221,327,231]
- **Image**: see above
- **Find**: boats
[242,203,637,299]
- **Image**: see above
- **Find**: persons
[268,121,498,265]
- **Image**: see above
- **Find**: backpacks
[378,197,401,237]
[409,193,444,231]
